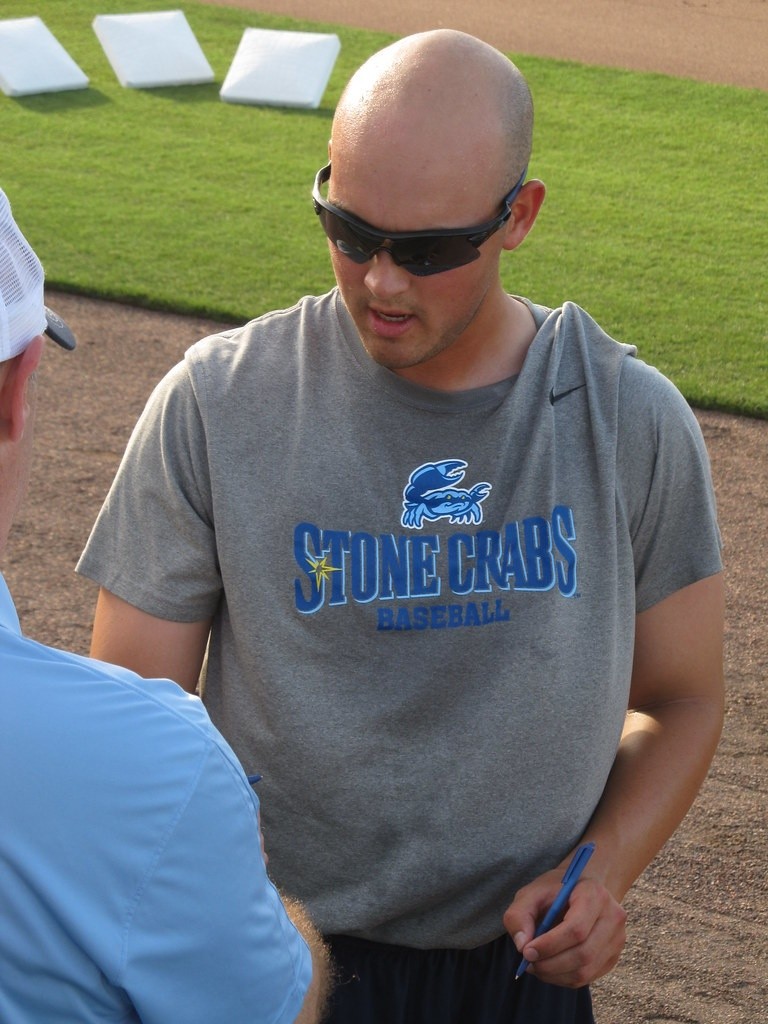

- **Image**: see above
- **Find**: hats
[0,185,77,362]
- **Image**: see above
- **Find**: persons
[0,188,328,1024]
[74,29,725,1023]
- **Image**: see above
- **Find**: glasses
[311,160,528,277]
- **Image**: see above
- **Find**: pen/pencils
[514,841,598,981]
